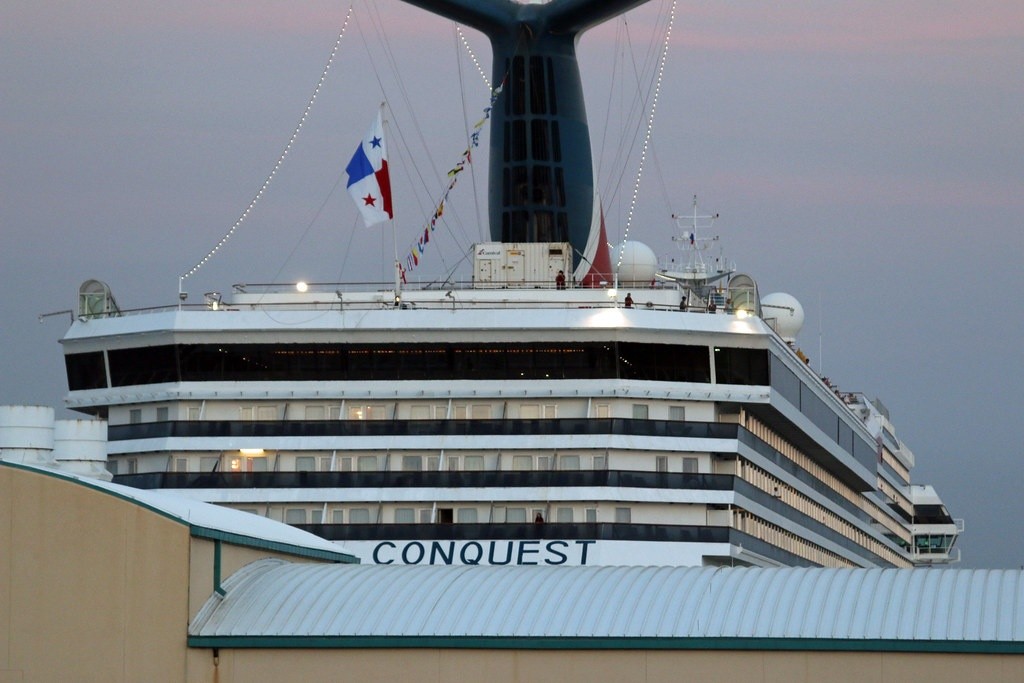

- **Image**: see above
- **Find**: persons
[556,270,567,290]
[725,298,734,314]
[709,300,716,312]
[679,296,687,311]
[625,293,634,308]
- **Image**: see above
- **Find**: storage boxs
[473,244,574,288]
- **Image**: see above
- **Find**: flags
[400,78,505,284]
[345,108,393,228]
